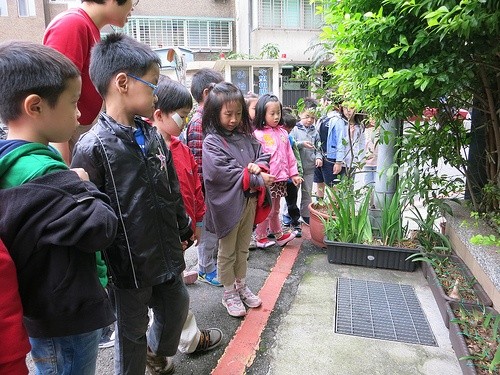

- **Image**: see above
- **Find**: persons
[43,0,137,349]
[142,69,223,354]
[0,241,32,375]
[0,42,116,375]
[201,81,277,317]
[72,32,195,375]
[244,91,379,261]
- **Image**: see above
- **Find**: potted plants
[310,183,423,271]
[291,66,371,246]
[426,254,493,327]
[445,300,500,375]
[413,229,455,276]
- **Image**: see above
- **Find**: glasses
[127,74,160,94]
[133,0,139,7]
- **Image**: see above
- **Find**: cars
[402,99,472,121]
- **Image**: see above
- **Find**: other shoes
[275,232,296,247]
[183,271,198,285]
[256,237,275,248]
[282,215,290,225]
[293,226,302,237]
[302,217,310,226]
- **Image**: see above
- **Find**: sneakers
[146,346,176,375]
[98,326,115,349]
[222,290,246,317]
[234,279,262,308]
[186,328,223,357]
[198,269,223,287]
